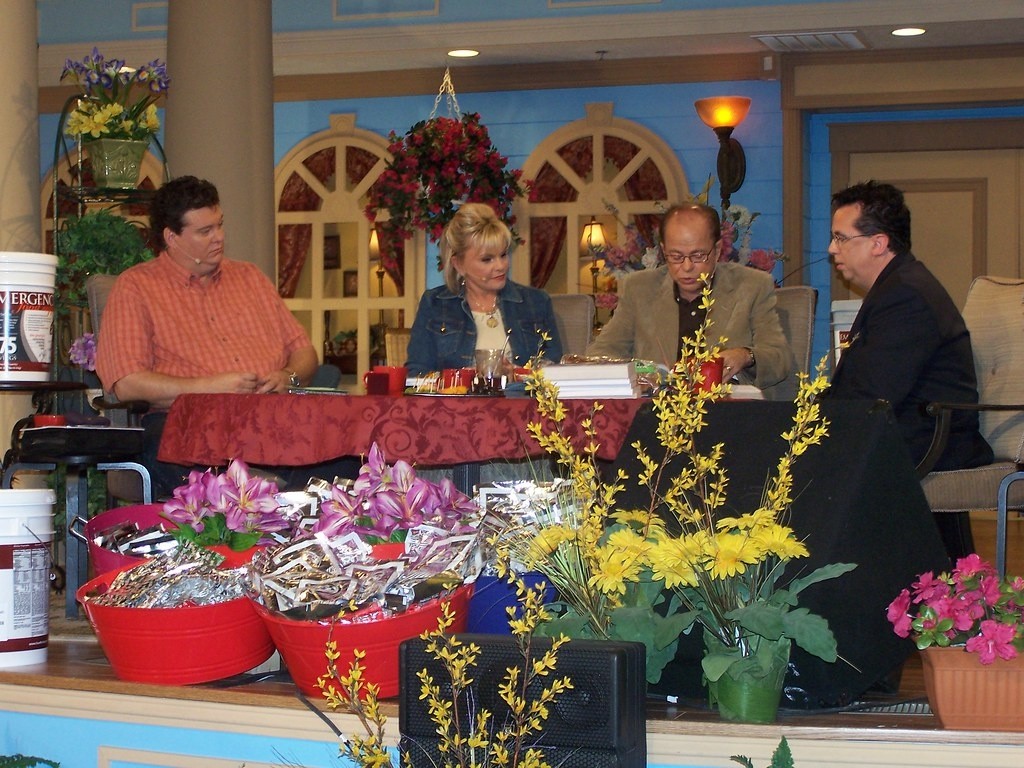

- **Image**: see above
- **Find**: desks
[156,389,764,500]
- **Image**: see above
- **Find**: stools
[0,393,157,619]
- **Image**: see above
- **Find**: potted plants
[324,324,383,376]
[50,206,155,365]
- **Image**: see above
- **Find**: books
[542,364,642,400]
[721,382,763,400]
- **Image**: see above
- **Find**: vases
[202,544,266,570]
[335,543,405,565]
[81,138,152,189]
[910,635,1024,732]
[702,626,791,722]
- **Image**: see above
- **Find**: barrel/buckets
[0,489,60,668]
[0,251,60,381]
[467,573,559,634]
[829,300,864,369]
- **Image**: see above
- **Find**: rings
[724,365,731,372]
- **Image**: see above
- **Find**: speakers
[397,632,647,768]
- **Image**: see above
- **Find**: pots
[68,503,191,577]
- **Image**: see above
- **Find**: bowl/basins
[254,544,472,700]
[76,545,276,685]
[366,536,407,563]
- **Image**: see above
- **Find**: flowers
[158,458,292,552]
[884,553,1024,666]
[67,332,96,371]
[361,111,535,276]
[59,46,172,197]
[293,251,860,768]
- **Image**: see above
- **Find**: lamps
[366,228,388,327]
[694,96,752,222]
[579,216,610,327]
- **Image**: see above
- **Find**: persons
[815,182,979,695]
[402,203,562,380]
[581,201,792,390]
[94,176,319,499]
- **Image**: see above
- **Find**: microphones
[704,276,713,285]
[479,276,488,282]
[192,257,201,263]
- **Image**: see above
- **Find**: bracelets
[744,346,756,368]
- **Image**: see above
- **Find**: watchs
[283,367,301,388]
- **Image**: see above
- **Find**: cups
[442,370,472,390]
[687,357,724,394]
[475,349,501,395]
[362,366,408,396]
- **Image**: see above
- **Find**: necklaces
[468,292,499,328]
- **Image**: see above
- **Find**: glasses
[826,233,870,245]
[662,249,713,265]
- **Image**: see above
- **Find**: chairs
[85,274,289,511]
[549,294,596,355]
[919,274,1024,585]
[760,286,818,401]
[384,327,413,367]
